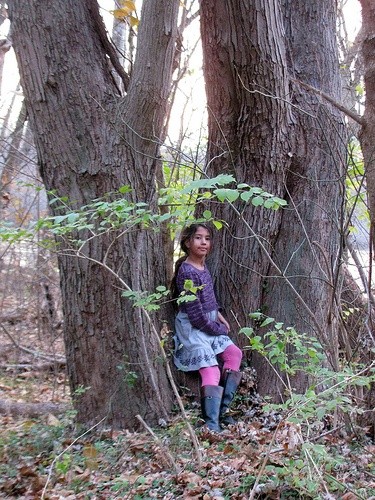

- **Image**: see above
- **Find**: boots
[218,368,243,425]
[200,385,224,432]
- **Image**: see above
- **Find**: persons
[167,222,247,437]
